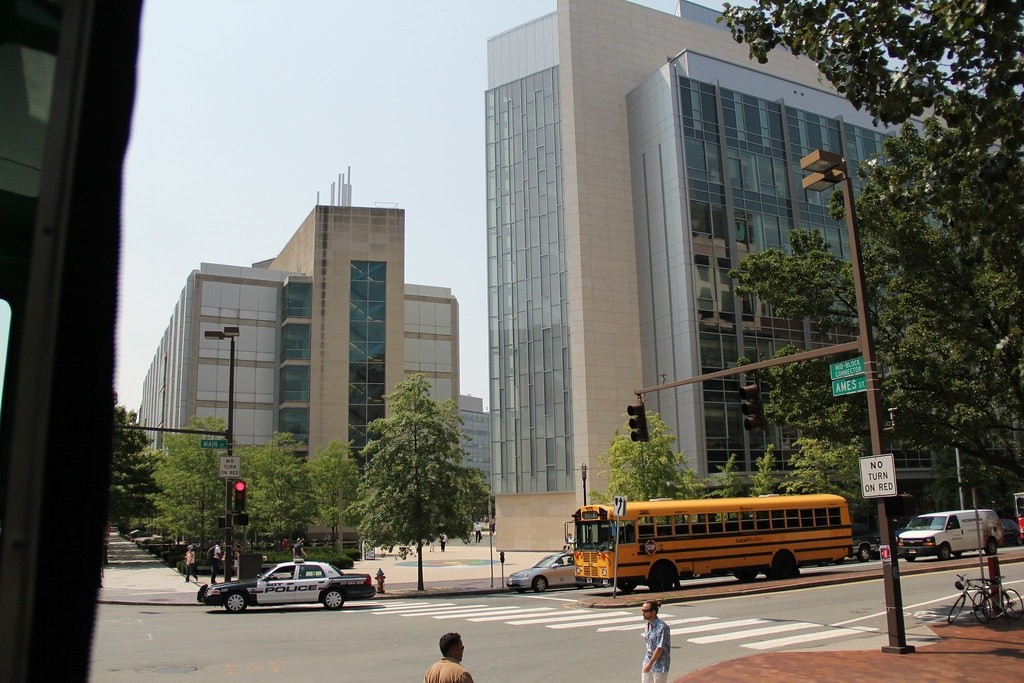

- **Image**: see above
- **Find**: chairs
[558,558,563,564]
[567,558,572,564]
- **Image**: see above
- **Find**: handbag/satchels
[480,536,482,539]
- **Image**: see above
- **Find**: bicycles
[947,574,1023,626]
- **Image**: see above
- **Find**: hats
[187,545,193,549]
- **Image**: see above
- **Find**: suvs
[1000,519,1023,546]
[851,522,876,563]
[897,508,1004,562]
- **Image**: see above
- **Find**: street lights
[203,326,240,581]
[798,148,916,655]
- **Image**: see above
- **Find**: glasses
[642,609,651,613]
[455,645,464,651]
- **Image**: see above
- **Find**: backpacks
[207,546,219,560]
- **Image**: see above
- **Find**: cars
[506,553,576,593]
[196,559,376,614]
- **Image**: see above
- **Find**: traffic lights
[739,380,766,432]
[626,402,648,443]
[234,481,246,512]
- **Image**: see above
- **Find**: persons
[640,600,670,683]
[567,533,574,549]
[422,632,474,683]
[183,534,331,584]
[429,514,495,553]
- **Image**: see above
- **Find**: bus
[563,492,853,592]
